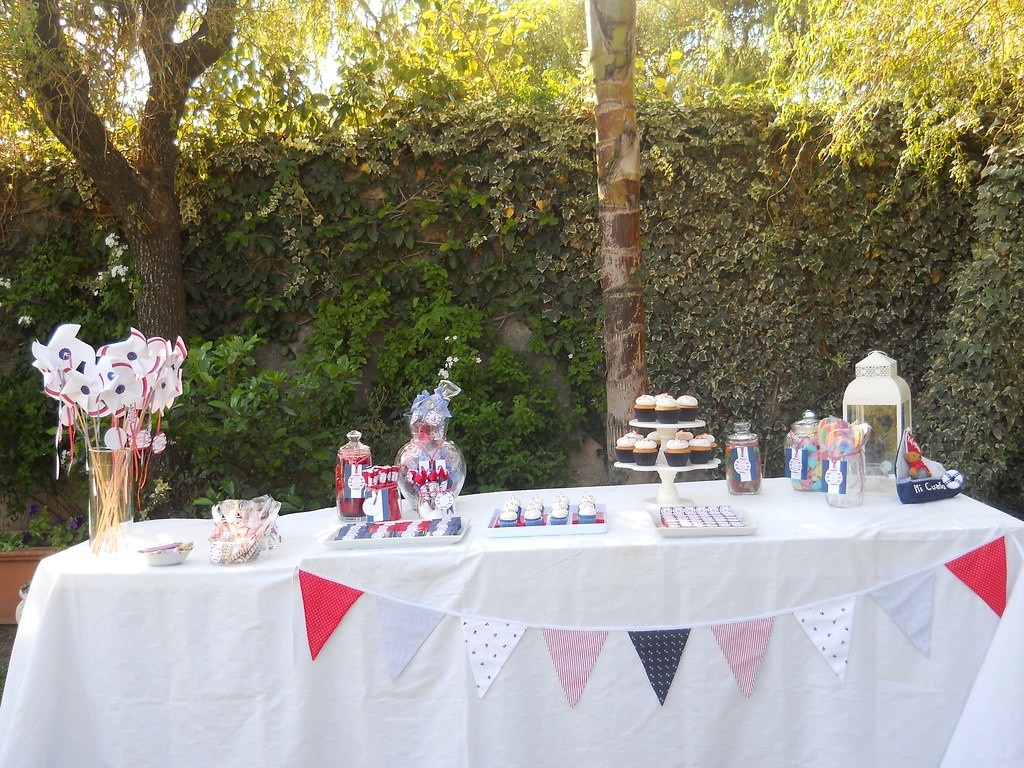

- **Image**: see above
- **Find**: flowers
[1,503,90,553]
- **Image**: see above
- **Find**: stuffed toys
[904,451,932,481]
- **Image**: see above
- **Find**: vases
[0,548,55,624]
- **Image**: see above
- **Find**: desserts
[633,393,698,423]
[498,491,596,527]
[614,430,717,466]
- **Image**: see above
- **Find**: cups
[827,450,866,508]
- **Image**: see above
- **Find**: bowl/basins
[138,547,191,566]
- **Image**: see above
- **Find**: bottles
[724,423,762,494]
[790,410,822,491]
[336,431,372,522]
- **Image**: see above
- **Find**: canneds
[726,420,762,495]
[336,430,372,521]
[784,410,826,491]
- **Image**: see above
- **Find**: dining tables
[0,472,1023,765]
